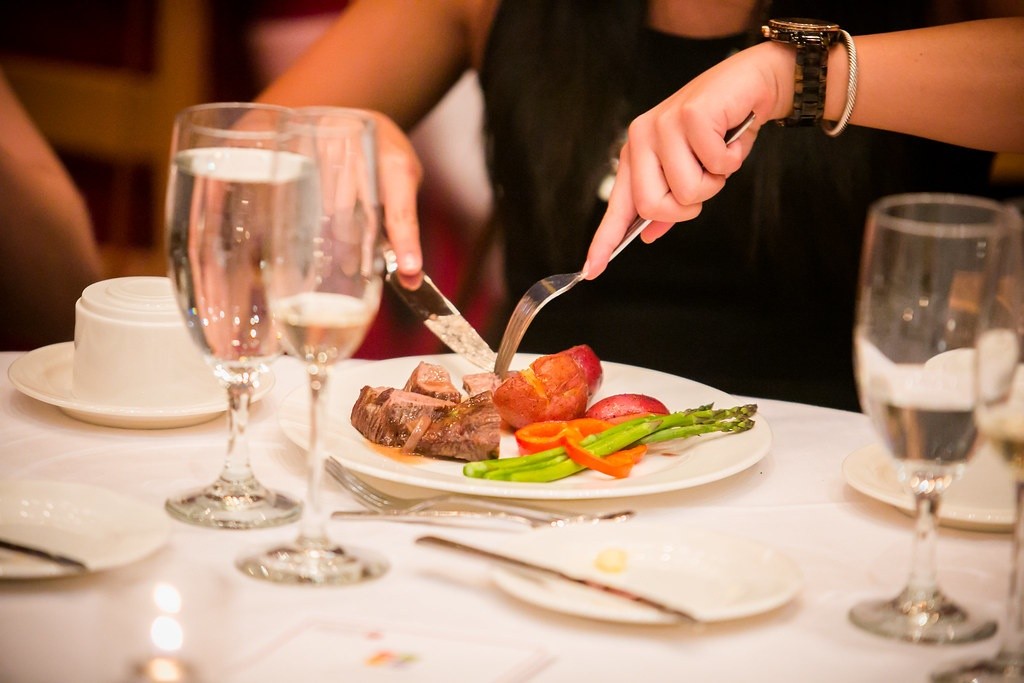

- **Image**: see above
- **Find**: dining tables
[0,357,1024,683]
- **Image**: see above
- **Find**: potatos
[492,354,590,426]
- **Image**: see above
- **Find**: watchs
[761,16,842,128]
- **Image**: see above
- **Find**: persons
[188,0,1024,412]
[0,83,101,352]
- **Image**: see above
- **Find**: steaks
[350,361,517,462]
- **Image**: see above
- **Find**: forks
[493,110,758,383]
[315,454,637,524]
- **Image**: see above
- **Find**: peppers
[515,417,648,477]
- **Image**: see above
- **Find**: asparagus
[463,403,757,481]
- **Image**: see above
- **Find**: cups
[71,273,219,415]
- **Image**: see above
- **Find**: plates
[843,439,1019,536]
[0,475,175,586]
[495,514,803,627]
[8,341,278,432]
[277,352,777,502]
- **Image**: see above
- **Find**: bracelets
[820,30,858,136]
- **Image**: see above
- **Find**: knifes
[377,233,498,372]
[417,535,707,632]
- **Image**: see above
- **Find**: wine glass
[163,100,321,529]
[926,196,1022,683]
[232,101,392,590]
[848,189,1023,643]
